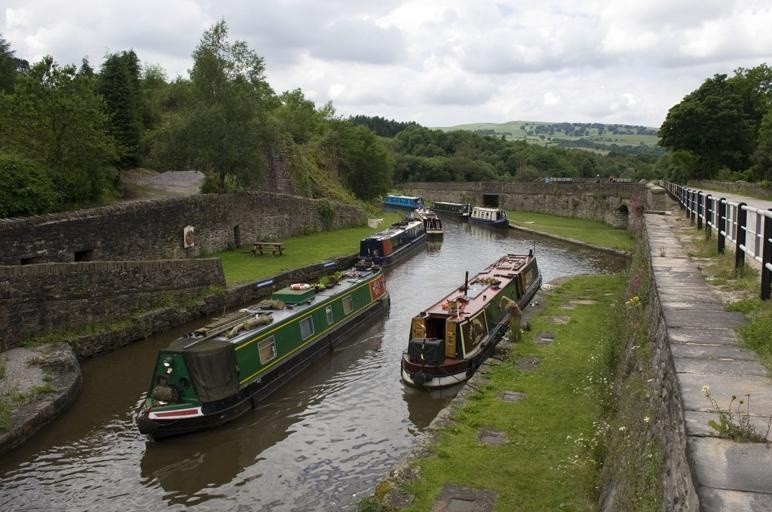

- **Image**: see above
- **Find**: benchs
[250,247,284,256]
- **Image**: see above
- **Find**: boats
[360,195,509,269]
[136,258,390,442]
[400,249,543,391]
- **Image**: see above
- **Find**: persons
[499,295,522,341]
[595,174,600,183]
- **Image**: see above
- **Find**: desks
[253,241,283,257]
